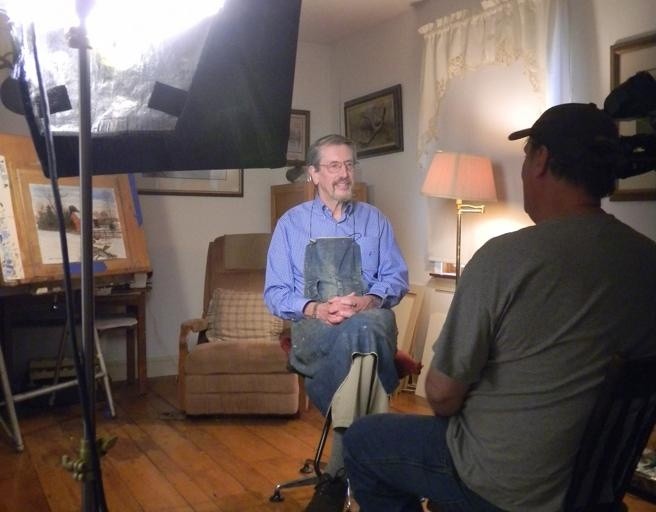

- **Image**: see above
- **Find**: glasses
[319,160,354,172]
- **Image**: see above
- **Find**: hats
[509,102,622,170]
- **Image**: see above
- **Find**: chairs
[560,356,655,511]
[175,233,309,417]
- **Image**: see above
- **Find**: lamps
[420,152,499,279]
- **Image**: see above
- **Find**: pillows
[202,287,284,344]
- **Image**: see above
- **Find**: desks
[1,285,152,399]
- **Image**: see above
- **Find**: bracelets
[311,301,321,318]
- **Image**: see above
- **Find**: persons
[340,102,656,512]
[262,133,412,510]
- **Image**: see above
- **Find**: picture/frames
[343,83,403,160]
[607,29,655,202]
[284,109,310,166]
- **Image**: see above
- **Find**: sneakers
[306,467,348,512]
